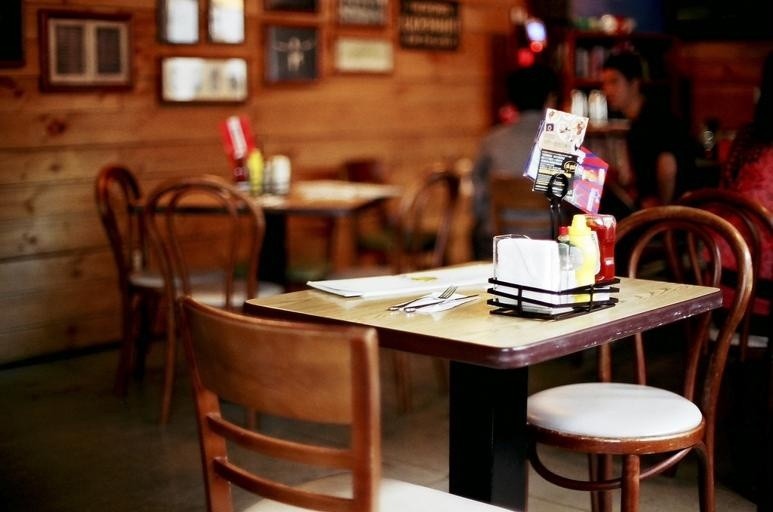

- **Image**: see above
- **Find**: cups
[494,234,528,309]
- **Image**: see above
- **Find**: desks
[241,256,727,509]
[129,172,406,292]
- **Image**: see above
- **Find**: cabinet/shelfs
[490,31,660,193]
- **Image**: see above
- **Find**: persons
[598,49,702,258]
[483,68,565,244]
[682,49,773,366]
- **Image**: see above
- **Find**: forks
[389,285,458,312]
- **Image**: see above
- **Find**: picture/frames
[262,21,320,86]
[325,32,396,76]
[37,8,136,92]
[395,0,461,51]
[158,57,249,104]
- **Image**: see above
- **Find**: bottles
[249,144,291,200]
[556,214,601,303]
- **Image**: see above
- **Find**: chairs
[97,164,225,384]
[140,173,269,447]
[524,200,758,512]
[171,293,517,512]
[339,163,444,262]
[356,168,465,407]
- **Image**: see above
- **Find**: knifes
[403,284,478,313]
